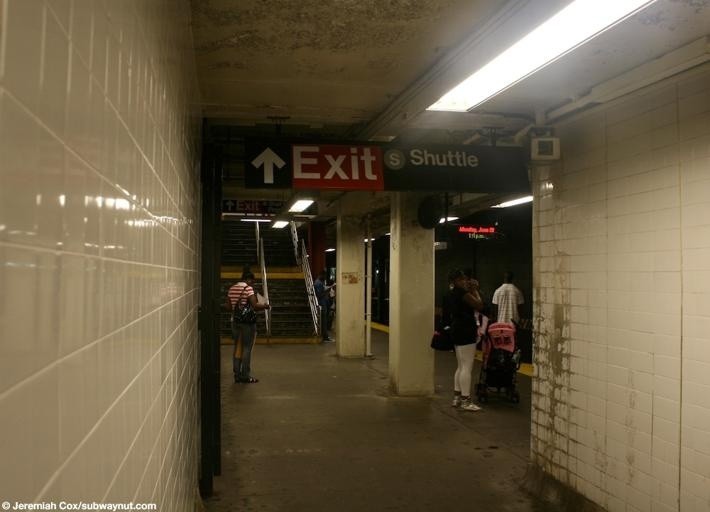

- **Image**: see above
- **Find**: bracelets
[265,305,270,309]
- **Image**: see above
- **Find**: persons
[314,270,336,343]
[491,270,526,328]
[223,270,273,383]
[442,268,484,412]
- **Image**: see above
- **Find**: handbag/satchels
[430,330,454,351]
[233,302,258,329]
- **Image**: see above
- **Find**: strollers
[474,322,520,404]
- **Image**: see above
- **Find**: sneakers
[235,376,259,383]
[323,337,336,343]
[451,399,482,411]
